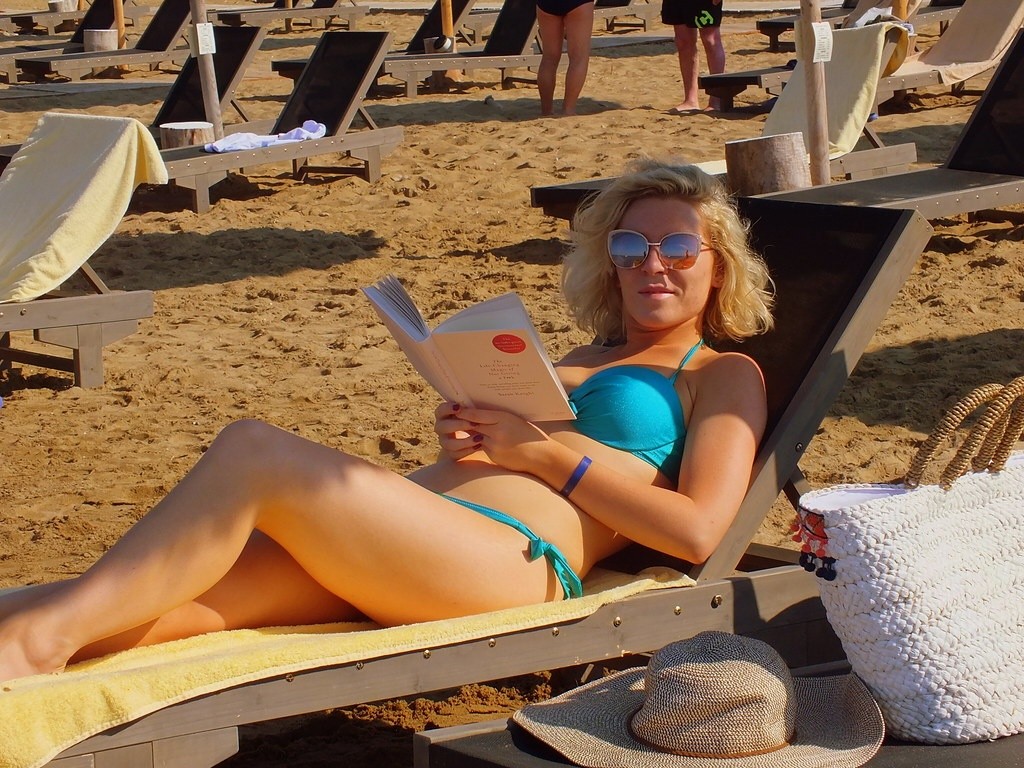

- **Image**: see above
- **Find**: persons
[536,0,595,115]
[0,158,775,682]
[661,0,725,115]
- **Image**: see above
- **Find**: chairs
[0,0,1024,768]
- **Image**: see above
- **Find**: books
[360,273,577,422]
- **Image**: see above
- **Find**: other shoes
[867,112,877,121]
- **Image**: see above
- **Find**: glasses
[606,229,717,270]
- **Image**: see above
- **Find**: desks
[725,131,813,201]
[83,29,119,73]
[48,0,79,31]
[160,122,215,150]
[424,37,458,78]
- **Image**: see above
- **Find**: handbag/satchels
[798,375,1024,744]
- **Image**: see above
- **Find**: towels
[840,0,922,29]
[204,119,327,153]
[760,22,910,164]
[0,111,169,304]
[889,0,1024,87]
[1,563,698,768]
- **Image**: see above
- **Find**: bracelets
[560,456,591,499]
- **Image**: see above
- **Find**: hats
[512,630,885,768]
